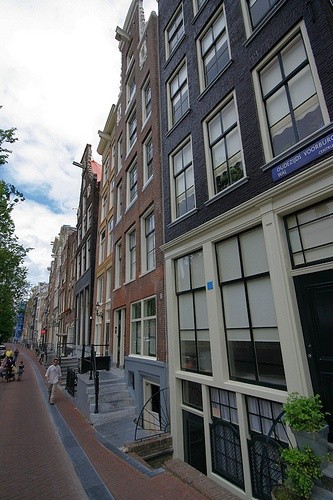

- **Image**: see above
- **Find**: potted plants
[282,391,330,461]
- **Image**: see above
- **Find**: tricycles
[0,365,16,382]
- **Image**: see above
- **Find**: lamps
[95,302,103,318]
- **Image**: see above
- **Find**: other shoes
[48,398,50,402]
[50,403,54,405]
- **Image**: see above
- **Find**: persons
[44,359,62,405]
[3,348,20,383]
[17,361,24,381]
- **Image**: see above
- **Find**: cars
[0,344,6,358]
